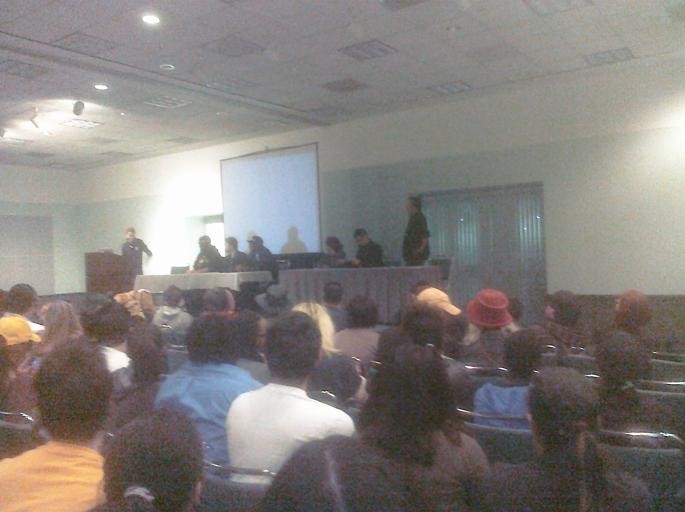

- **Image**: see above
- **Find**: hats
[466,289,512,329]
[254,284,296,314]
[0,315,42,347]
[246,235,263,244]
[545,289,581,316]
[417,286,462,317]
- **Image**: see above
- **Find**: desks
[82,252,136,297]
[132,271,273,311]
[278,268,442,326]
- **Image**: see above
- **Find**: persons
[503,292,524,322]
[219,237,247,273]
[247,235,279,271]
[400,197,430,265]
[400,302,472,413]
[121,227,152,276]
[0,334,117,511]
[0,314,43,412]
[350,228,382,267]
[528,288,586,349]
[225,311,355,484]
[462,290,519,353]
[37,300,84,345]
[193,235,218,272]
[153,311,264,478]
[320,284,343,306]
[259,437,421,511]
[361,338,492,509]
[474,327,541,430]
[473,365,653,508]
[601,289,653,332]
[293,301,369,410]
[202,289,240,322]
[74,303,142,396]
[93,408,206,511]
[591,328,685,449]
[318,237,350,268]
[153,286,189,335]
[333,297,381,355]
[7,281,37,317]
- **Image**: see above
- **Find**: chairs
[646,357,683,380]
[637,378,683,391]
[158,322,179,345]
[573,429,594,475]
[541,343,557,353]
[454,406,538,461]
[629,391,684,427]
[427,256,452,285]
[463,363,509,378]
[331,353,364,379]
[595,430,684,504]
[651,350,683,357]
[370,360,382,373]
[2,410,47,455]
[569,347,587,354]
[200,459,276,511]
[163,344,192,374]
[309,390,336,407]
